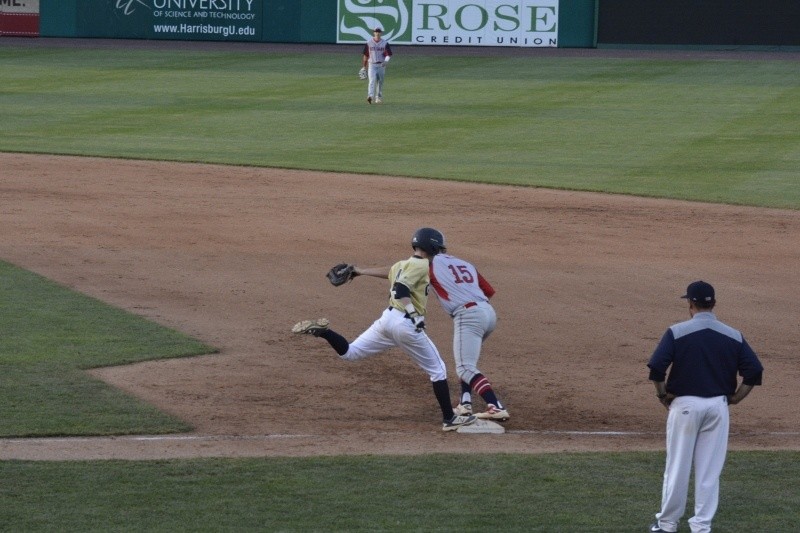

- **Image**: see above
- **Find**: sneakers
[648,522,671,533]
[442,416,477,432]
[472,404,510,422]
[452,403,472,416]
[292,318,330,334]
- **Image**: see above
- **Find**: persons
[362,28,392,104]
[647,281,764,533]
[291,228,477,431]
[348,246,510,421]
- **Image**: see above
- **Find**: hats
[373,28,383,32]
[680,280,715,302]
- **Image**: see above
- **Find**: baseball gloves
[326,260,360,288]
[358,66,368,80]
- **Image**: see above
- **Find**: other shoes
[367,96,373,104]
[375,98,382,104]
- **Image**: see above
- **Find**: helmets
[411,228,447,251]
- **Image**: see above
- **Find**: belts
[388,305,411,319]
[465,302,477,308]
[372,62,382,64]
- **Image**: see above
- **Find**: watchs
[656,394,666,399]
